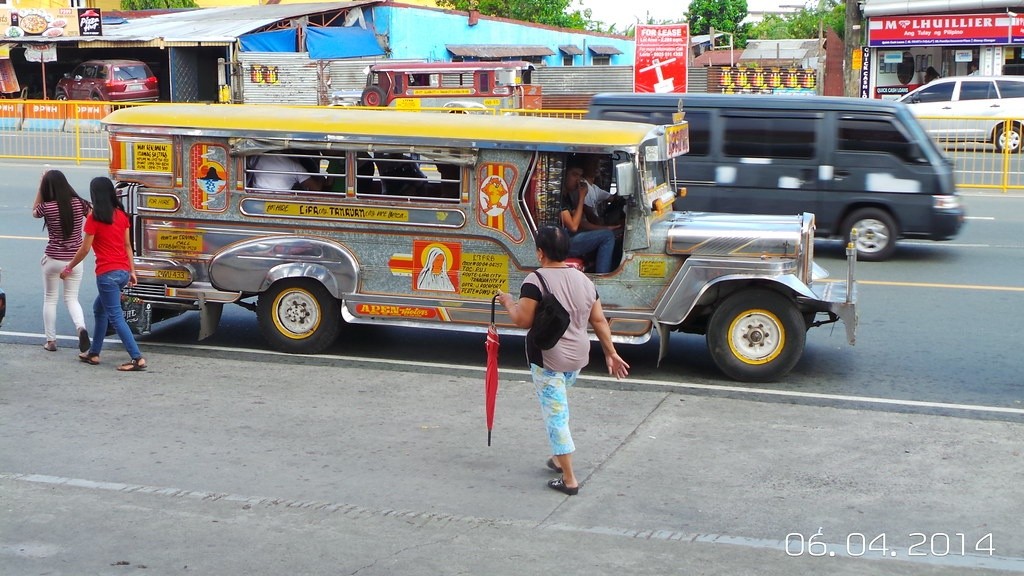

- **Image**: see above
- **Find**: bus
[361,59,535,118]
[101,106,862,383]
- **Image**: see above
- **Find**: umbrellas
[485,295,500,446]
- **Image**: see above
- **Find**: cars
[892,76,1024,153]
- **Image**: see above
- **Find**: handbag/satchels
[529,270,571,350]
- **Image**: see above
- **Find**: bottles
[253,70,276,82]
[720,72,814,88]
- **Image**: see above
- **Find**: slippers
[548,473,578,495]
[547,458,562,473]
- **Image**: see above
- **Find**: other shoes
[44,341,57,351]
[78,327,90,352]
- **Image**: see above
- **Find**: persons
[561,155,623,273]
[925,66,940,84]
[497,222,629,495]
[60,176,147,372]
[32,169,94,352]
[968,62,979,76]
[245,149,460,197]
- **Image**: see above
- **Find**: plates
[4,26,24,37]
[42,27,63,37]
[48,18,66,27]
[20,14,47,34]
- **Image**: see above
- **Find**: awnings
[447,45,556,57]
[560,45,583,55]
[591,46,623,55]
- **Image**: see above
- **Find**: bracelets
[65,266,72,273]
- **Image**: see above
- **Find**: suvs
[54,59,158,109]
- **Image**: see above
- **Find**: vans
[588,93,963,261]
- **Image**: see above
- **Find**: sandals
[117,355,147,370]
[79,349,99,365]
